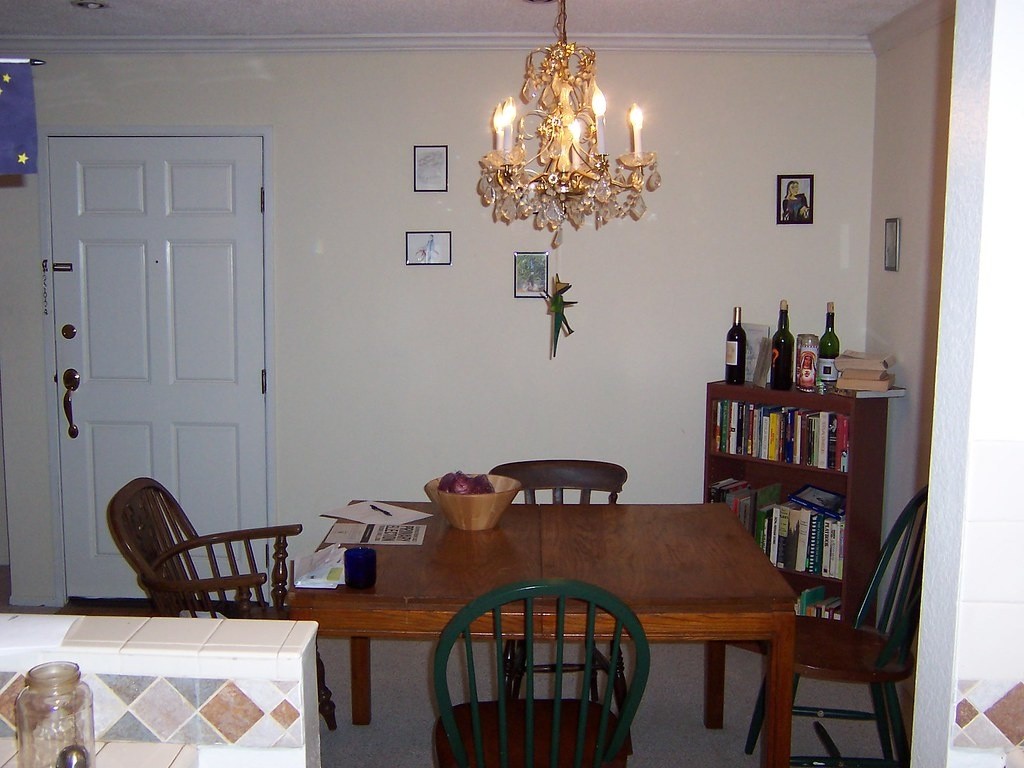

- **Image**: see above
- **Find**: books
[708,476,846,580]
[794,585,841,622]
[835,348,907,398]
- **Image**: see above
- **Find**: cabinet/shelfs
[704,378,887,729]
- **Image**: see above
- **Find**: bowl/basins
[424,474,522,531]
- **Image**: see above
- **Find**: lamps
[475,0,661,249]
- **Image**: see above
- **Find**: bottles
[770,300,795,391]
[796,334,820,392]
[819,302,839,387]
[14,661,96,768]
[726,307,746,385]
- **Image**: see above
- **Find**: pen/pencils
[370,505,393,516]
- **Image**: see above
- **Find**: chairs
[487,459,634,755]
[106,477,338,731]
[744,485,928,764]
[433,581,650,768]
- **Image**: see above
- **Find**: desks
[287,500,794,768]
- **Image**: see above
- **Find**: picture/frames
[406,231,451,266]
[413,145,448,193]
[514,251,549,298]
[884,218,900,272]
[776,174,815,225]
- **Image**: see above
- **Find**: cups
[344,547,376,590]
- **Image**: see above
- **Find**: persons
[714,399,850,474]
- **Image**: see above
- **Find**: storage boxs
[834,350,896,392]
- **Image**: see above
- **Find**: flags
[0,62,39,177]
